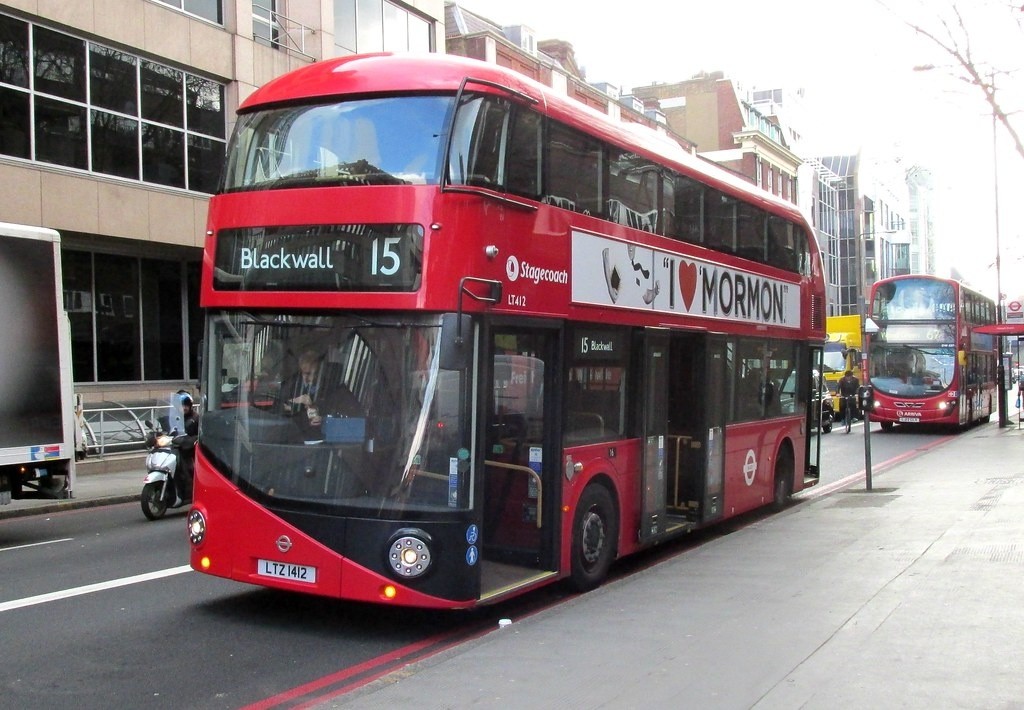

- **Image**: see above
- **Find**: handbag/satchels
[1015,395,1020,408]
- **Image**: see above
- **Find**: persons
[281,349,333,424]
[196,382,206,412]
[1018,373,1024,410]
[836,371,859,426]
[147,390,199,445]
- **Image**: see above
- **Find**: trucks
[817,313,862,421]
[0,221,76,502]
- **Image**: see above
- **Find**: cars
[1011,365,1024,384]
[773,369,835,433]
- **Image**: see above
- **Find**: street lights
[913,62,1009,430]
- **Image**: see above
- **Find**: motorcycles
[141,392,193,521]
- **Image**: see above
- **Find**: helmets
[173,390,194,407]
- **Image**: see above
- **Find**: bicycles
[837,393,857,433]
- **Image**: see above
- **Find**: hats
[845,370,854,377]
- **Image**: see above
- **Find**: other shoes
[841,418,845,424]
[852,418,858,423]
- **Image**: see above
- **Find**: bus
[187,54,826,609]
[866,274,998,433]
